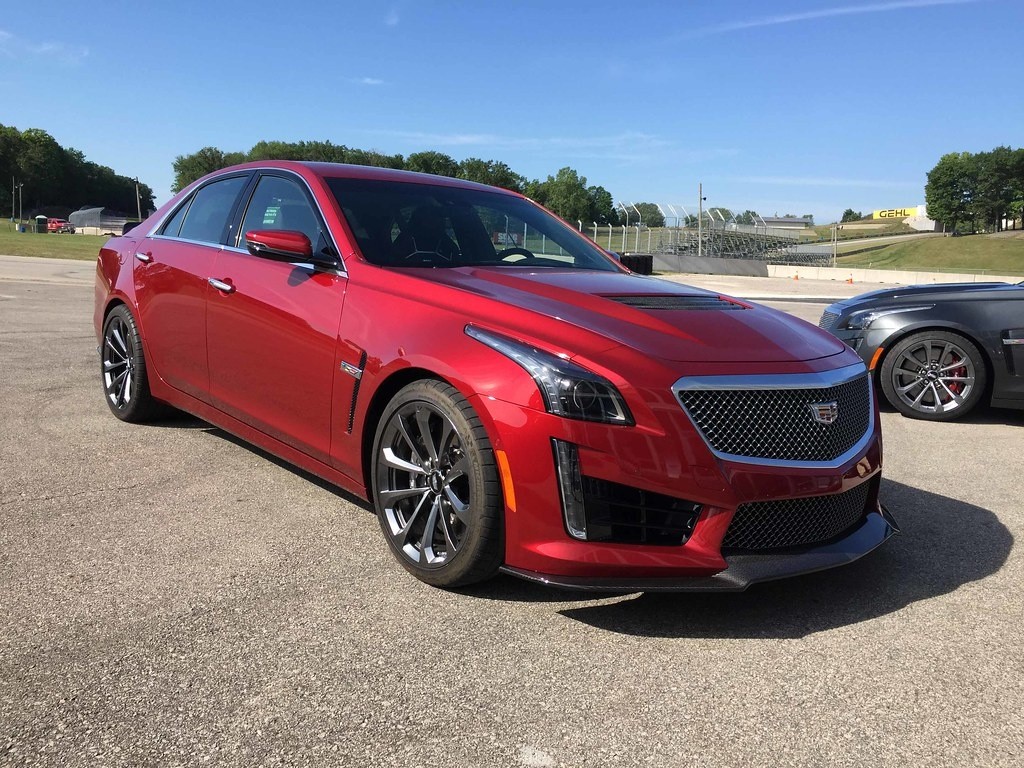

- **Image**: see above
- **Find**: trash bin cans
[10,217,14,222]
[35,215,48,233]
[21,225,25,232]
[16,223,19,231]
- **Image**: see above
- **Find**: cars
[91,160,902,593]
[48,218,70,233]
[819,278,1024,423]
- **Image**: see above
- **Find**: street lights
[133,176,142,222]
[15,181,24,223]
[698,182,707,256]
[833,223,843,268]
